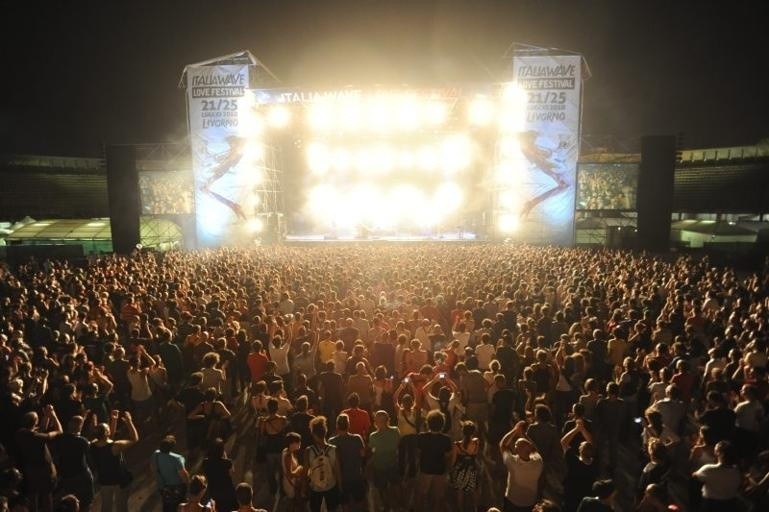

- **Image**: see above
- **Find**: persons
[139,173,196,215]
[577,164,639,211]
[0,238,769,512]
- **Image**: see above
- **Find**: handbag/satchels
[116,460,134,489]
[379,390,394,410]
[160,484,185,505]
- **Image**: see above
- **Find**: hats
[577,496,602,512]
[591,479,616,496]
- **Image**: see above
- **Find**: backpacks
[440,402,452,433]
[309,445,334,492]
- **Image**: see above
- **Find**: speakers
[637,134,674,251]
[105,144,141,254]
